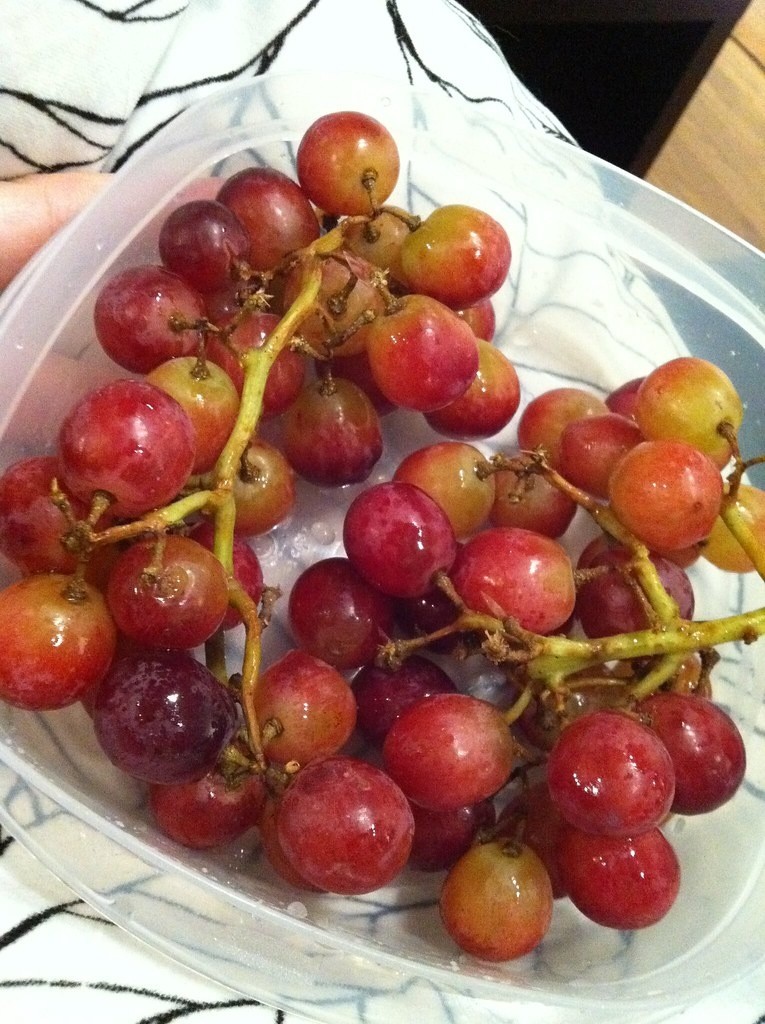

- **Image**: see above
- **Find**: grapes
[0,111,765,964]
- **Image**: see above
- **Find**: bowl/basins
[0,75,764,1024]
